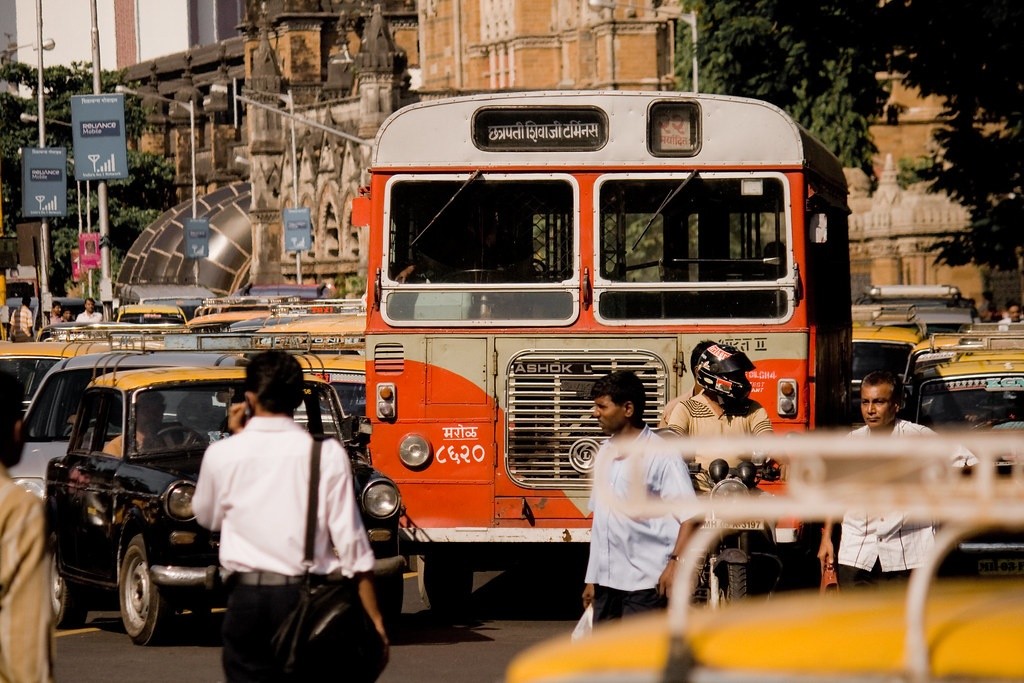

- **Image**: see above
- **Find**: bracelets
[668,553,679,560]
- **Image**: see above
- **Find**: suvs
[230,282,332,304]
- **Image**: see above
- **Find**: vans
[114,282,215,320]
[7,296,102,328]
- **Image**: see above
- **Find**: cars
[505,282,1024,683]
[0,300,403,646]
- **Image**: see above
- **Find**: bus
[350,90,855,543]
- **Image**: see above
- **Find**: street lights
[19,111,94,300]
[587,0,699,93]
[208,83,303,286]
[115,84,198,289]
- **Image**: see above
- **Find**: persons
[192,350,390,683]
[103,390,188,457]
[817,370,942,589]
[86,241,94,254]
[765,242,786,279]
[76,298,103,321]
[659,342,794,604]
[582,371,704,627]
[10,297,34,342]
[50,301,74,324]
[390,218,528,281]
[0,370,57,683]
[979,291,1024,324]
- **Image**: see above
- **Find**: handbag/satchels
[572,603,595,639]
[270,581,383,683]
[821,556,840,597]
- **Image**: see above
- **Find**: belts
[239,570,303,588]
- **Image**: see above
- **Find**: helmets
[698,346,754,400]
